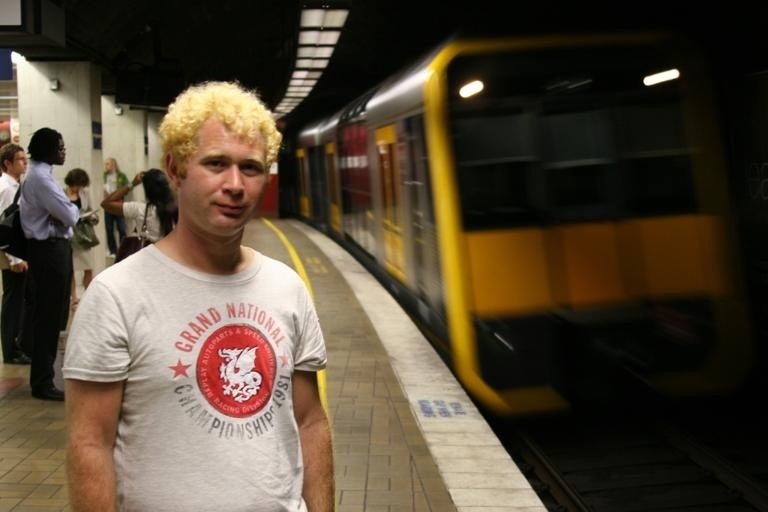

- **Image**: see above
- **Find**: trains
[286,20,758,437]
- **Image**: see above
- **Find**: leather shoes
[30,386,64,401]
[3,353,33,365]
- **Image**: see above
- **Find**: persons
[0,142,31,366]
[98,166,179,250]
[58,79,339,511]
[62,167,103,318]
[17,127,69,402]
[101,157,130,259]
[0,250,29,273]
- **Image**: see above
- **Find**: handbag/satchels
[0,201,26,252]
[113,236,144,265]
[75,219,99,250]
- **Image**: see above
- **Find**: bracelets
[128,182,133,190]
